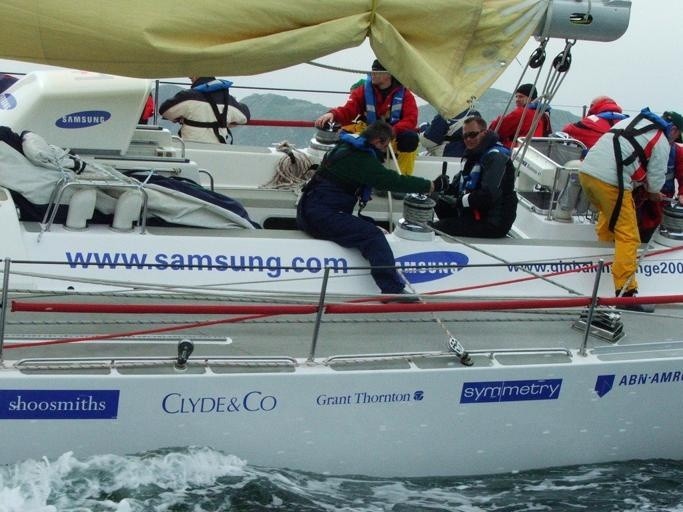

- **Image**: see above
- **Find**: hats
[372,59,387,71]
[590,96,616,109]
[516,84,537,99]
[662,111,683,143]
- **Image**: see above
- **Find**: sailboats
[0,1,682,478]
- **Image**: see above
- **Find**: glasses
[463,130,484,138]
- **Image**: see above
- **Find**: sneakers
[615,295,656,312]
[382,290,419,302]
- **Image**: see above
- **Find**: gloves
[438,194,458,206]
[432,175,450,191]
[419,122,430,132]
[651,192,667,202]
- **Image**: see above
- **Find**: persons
[314,59,419,200]
[424,114,519,239]
[295,121,450,304]
[487,83,553,151]
[578,106,683,313]
[159,76,251,145]
[554,95,630,183]
[416,107,482,157]
[660,135,683,208]
